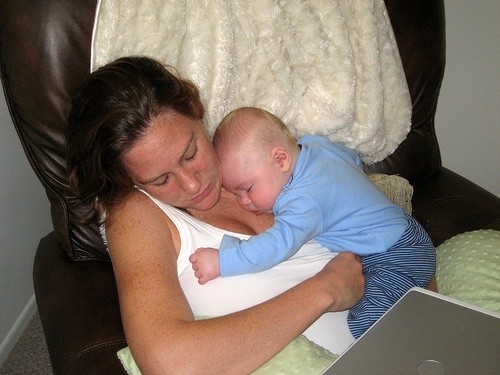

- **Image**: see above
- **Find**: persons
[189,106,437,340]
[65,56,436,375]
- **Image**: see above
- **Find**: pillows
[115,226,500,375]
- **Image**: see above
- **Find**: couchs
[0,0,500,375]
